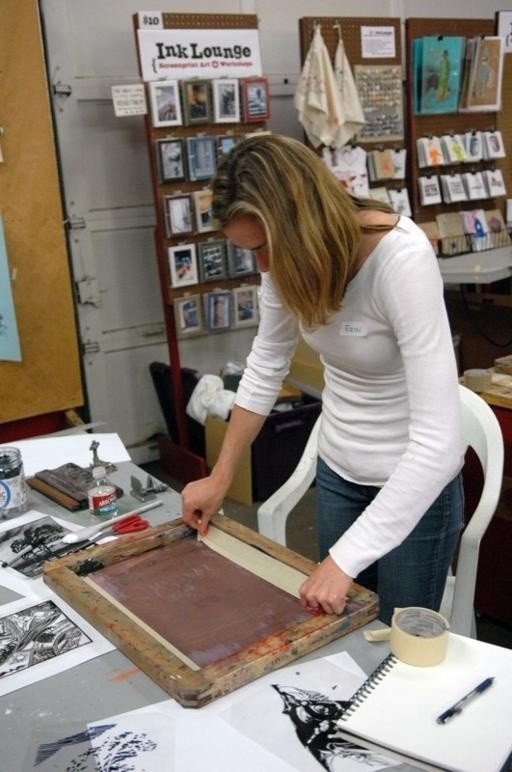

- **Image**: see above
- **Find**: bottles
[0,446,28,520]
[88,466,118,524]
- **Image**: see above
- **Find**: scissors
[73,514,149,553]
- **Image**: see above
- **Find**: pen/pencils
[437,676,494,725]
[2,535,48,568]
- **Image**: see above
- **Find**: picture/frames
[42,511,380,709]
[148,76,271,340]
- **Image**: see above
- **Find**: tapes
[388,606,450,668]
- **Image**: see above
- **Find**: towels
[330,39,366,150]
[294,25,347,149]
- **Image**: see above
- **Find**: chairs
[256,384,504,639]
[149,361,206,461]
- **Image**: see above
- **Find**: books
[482,355,512,409]
[335,631,512,772]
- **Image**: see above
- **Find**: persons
[180,133,468,629]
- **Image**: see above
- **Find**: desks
[446,366,512,635]
[222,375,322,501]
[0,428,452,772]
